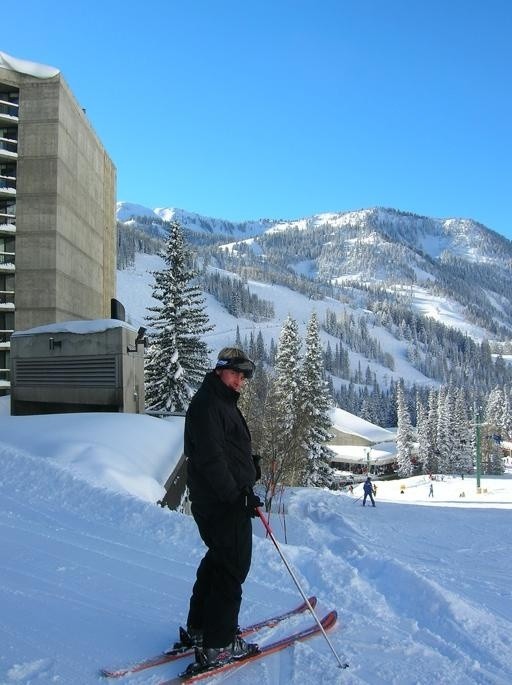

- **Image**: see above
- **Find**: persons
[429,484,433,497]
[349,484,353,495]
[185,348,262,668]
[363,477,376,507]
[373,484,377,496]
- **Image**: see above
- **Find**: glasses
[216,357,256,380]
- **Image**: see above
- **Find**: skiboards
[99,597,337,684]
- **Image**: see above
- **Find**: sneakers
[202,637,252,665]
[189,624,241,645]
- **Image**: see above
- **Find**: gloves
[236,487,264,519]
